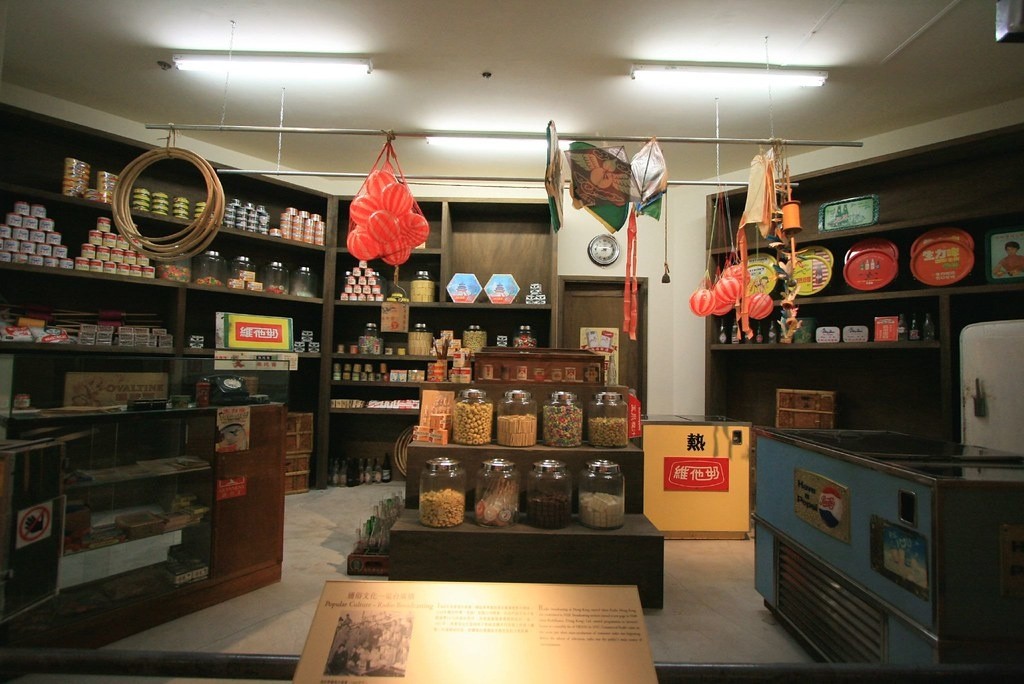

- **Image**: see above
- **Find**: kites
[545,120,668,341]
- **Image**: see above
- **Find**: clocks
[588,233,621,266]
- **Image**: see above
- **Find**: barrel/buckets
[409,280,435,302]
[462,330,487,361]
[407,332,433,355]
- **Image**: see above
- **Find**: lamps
[630,64,828,87]
[172,54,373,75]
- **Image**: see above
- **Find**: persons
[323,617,413,678]
[993,241,1024,277]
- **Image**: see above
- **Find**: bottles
[923,313,935,342]
[331,453,391,488]
[717,318,729,344]
[452,389,494,446]
[909,312,921,341]
[418,457,468,529]
[415,270,431,280]
[468,325,482,332]
[519,325,531,336]
[364,323,378,338]
[526,459,573,530]
[474,458,521,529]
[754,320,765,344]
[578,458,626,530]
[156,244,192,284]
[587,392,629,449]
[496,389,538,448]
[768,321,777,343]
[229,256,257,290]
[731,318,740,344]
[415,323,427,332]
[897,314,908,341]
[258,262,290,296]
[291,266,318,298]
[192,250,228,287]
[542,391,583,449]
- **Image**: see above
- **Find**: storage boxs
[874,316,899,341]
[283,454,309,495]
[348,547,389,576]
[286,412,313,454]
[446,273,483,303]
[775,387,841,428]
[484,274,520,305]
[63,371,169,407]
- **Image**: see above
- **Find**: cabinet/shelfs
[0,401,287,684]
[389,380,664,609]
[704,122,1024,532]
[0,101,558,489]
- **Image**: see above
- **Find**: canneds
[340,259,384,302]
[62,158,207,221]
[516,365,528,381]
[384,347,406,355]
[450,352,472,384]
[0,201,74,269]
[75,216,155,278]
[333,363,390,382]
[482,365,493,379]
[533,368,545,381]
[221,199,326,247]
[551,369,562,382]
[337,344,358,354]
[501,366,511,380]
